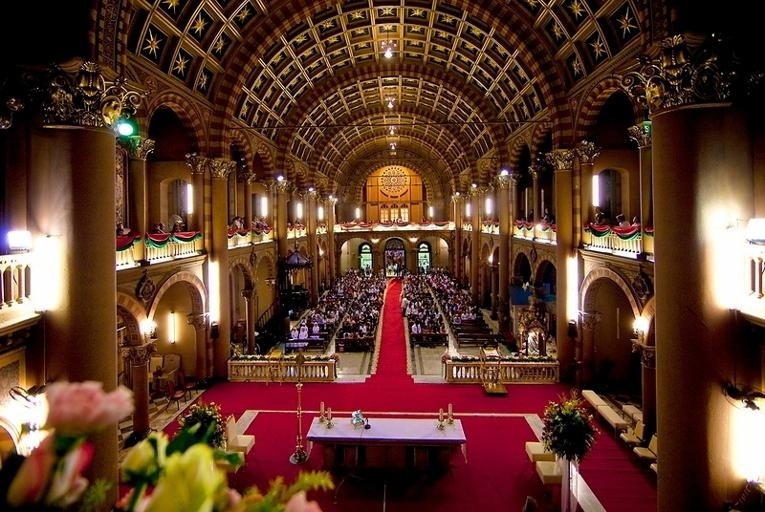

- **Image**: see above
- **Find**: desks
[306,411,467,483]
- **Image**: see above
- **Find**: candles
[439,403,452,421]
[320,402,332,420]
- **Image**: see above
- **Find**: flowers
[0,375,334,512]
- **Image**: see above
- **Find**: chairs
[211,413,256,476]
[146,353,198,411]
[618,422,658,475]
[523,435,576,486]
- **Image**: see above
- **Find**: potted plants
[541,394,601,512]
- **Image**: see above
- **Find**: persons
[526,209,535,223]
[629,215,641,225]
[542,207,555,224]
[158,223,165,230]
[152,223,163,232]
[616,213,631,227]
[116,222,127,236]
[172,223,181,231]
[594,206,610,224]
[230,213,305,230]
[282,262,495,347]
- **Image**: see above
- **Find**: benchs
[405,267,499,349]
[284,267,386,353]
[582,388,643,429]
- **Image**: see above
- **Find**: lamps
[380,37,399,156]
[169,311,175,344]
